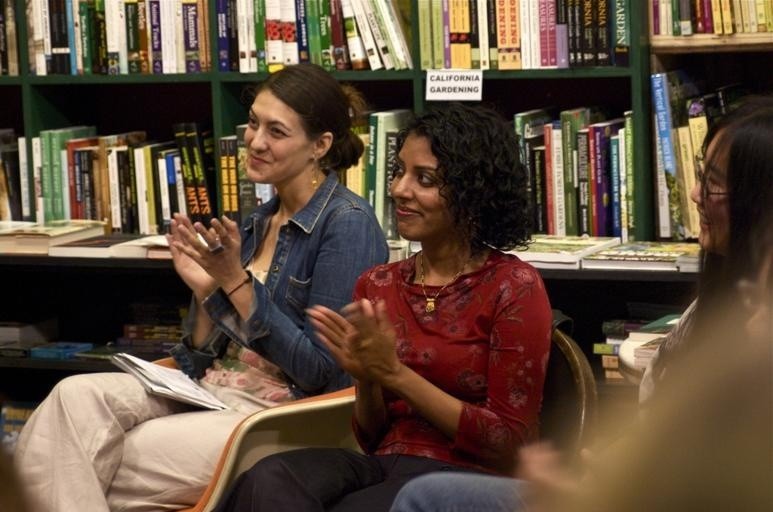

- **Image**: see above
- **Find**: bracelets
[223,277,252,298]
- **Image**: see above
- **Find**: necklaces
[421,251,472,312]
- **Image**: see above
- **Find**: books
[1,303,186,457]
[592,301,684,380]
[651,0,773,36]
[111,352,229,411]
[335,109,410,242]
[649,69,742,239]
[503,234,700,272]
[221,124,274,228]
[513,107,635,243]
[0,124,217,258]
[0,0,631,76]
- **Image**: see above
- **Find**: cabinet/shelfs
[529,329,601,483]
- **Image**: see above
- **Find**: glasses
[693,153,729,201]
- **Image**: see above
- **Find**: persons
[235,101,553,511]
[388,94,771,512]
[10,63,391,511]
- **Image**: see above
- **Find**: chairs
[134,376,357,512]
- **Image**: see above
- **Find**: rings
[354,345,363,353]
